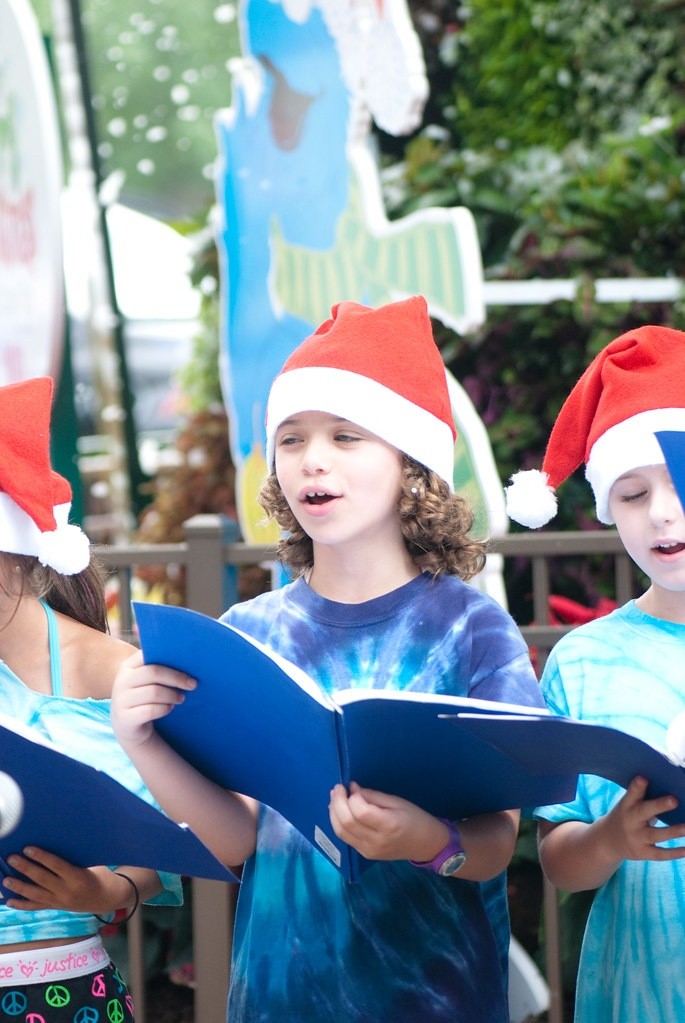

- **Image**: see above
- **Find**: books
[0,713,242,905]
[130,601,579,889]
[438,712,685,829]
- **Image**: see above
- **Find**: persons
[504,324,685,1023]
[0,377,184,1023]
[111,296,546,1023]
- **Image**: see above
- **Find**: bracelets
[93,872,139,926]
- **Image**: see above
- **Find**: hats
[0,376,90,575]
[265,295,457,496]
[505,325,685,525]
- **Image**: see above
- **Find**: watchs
[407,818,466,877]
